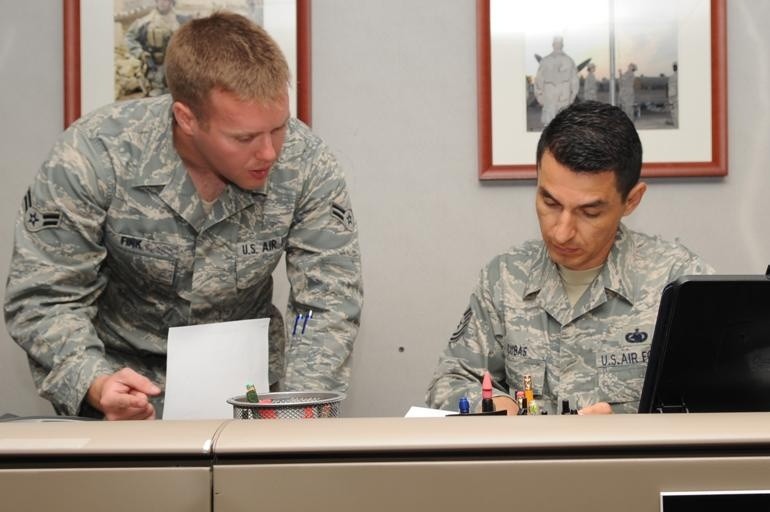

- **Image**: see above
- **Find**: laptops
[638,274,770,412]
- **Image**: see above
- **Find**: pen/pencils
[291,310,313,338]
[516,374,540,416]
[460,397,471,414]
[481,371,494,415]
[242,384,333,418]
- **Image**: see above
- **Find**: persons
[535,35,580,129]
[667,62,678,126]
[584,64,600,104]
[617,62,638,120]
[426,99,727,415]
[125,1,196,93]
[2,12,364,420]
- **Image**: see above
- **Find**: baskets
[227,391,346,418]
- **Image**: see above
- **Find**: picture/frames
[60,0,315,131]
[472,0,729,185]
